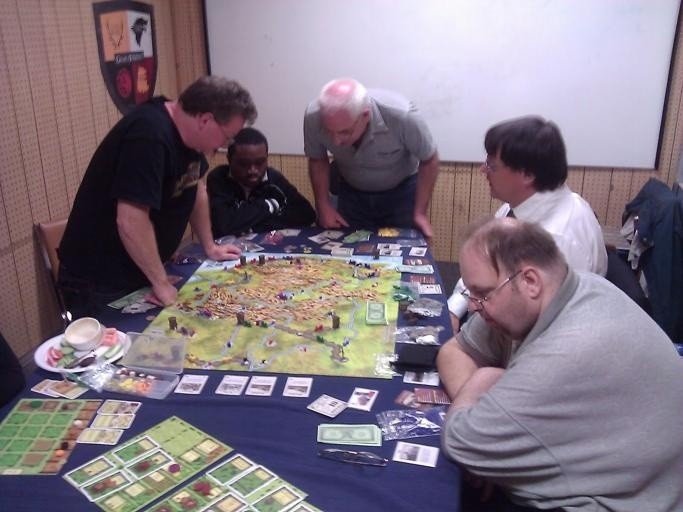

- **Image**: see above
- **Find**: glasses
[461,270,522,312]
[327,118,361,136]
[221,126,235,146]
[484,160,508,175]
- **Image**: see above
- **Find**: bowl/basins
[64,316,104,352]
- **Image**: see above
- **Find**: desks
[0,224,464,512]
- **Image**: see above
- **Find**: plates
[33,329,132,374]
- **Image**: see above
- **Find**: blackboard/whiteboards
[201,0,683,172]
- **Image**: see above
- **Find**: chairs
[606,243,651,316]
[37,216,70,325]
